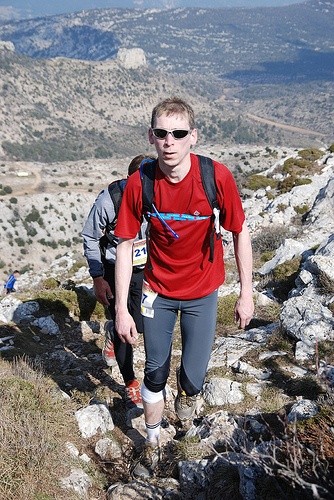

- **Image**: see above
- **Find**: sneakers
[174,366,201,421]
[102,320,117,366]
[132,443,162,479]
[124,380,143,409]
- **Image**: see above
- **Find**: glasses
[151,127,192,139]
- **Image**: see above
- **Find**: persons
[115,98,255,480]
[80,154,158,410]
[4,270,19,295]
